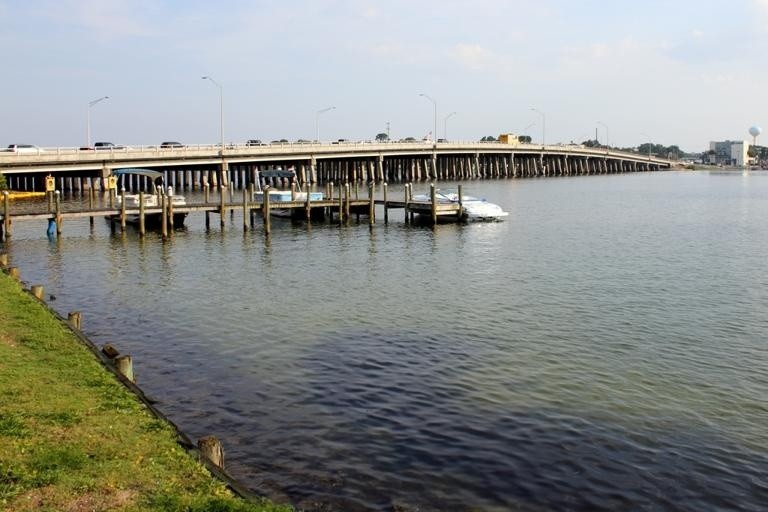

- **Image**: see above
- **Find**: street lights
[529,107,545,150]
[86,95,111,148]
[444,111,457,140]
[597,120,610,151]
[315,106,338,140]
[201,75,225,151]
[419,93,437,142]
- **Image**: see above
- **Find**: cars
[79,141,133,150]
[244,136,449,146]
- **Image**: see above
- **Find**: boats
[253,165,327,219]
[105,167,189,228]
[411,190,508,223]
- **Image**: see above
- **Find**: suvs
[7,144,44,152]
[160,142,188,148]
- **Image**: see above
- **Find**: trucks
[499,133,523,146]
